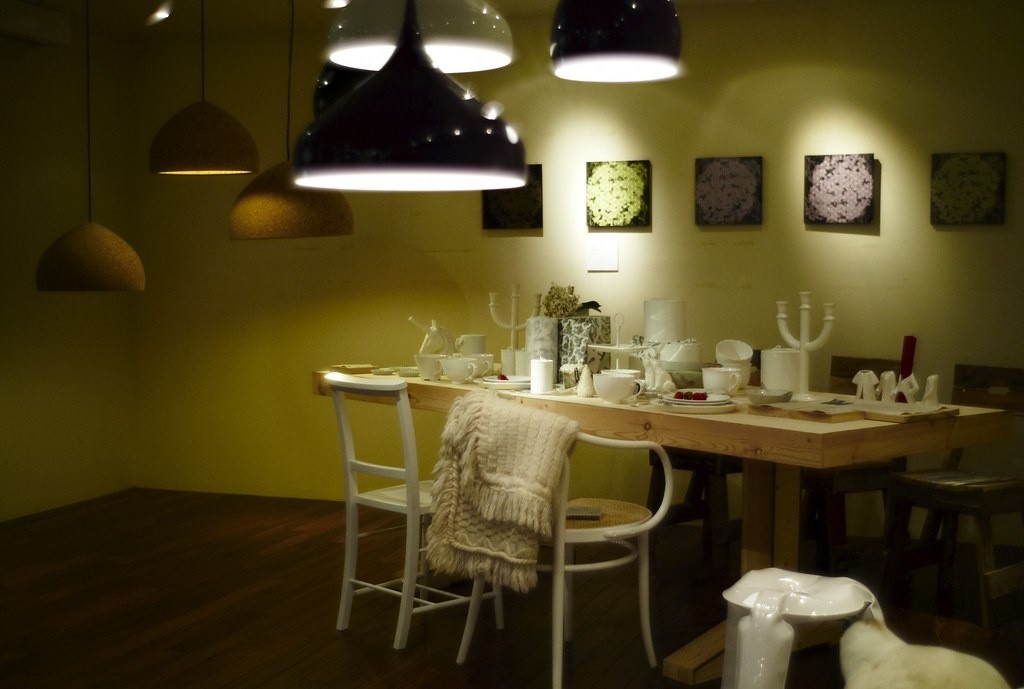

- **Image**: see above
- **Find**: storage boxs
[557,316,611,383]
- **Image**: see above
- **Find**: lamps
[228,0,353,242]
[318,0,515,76]
[149,0,260,177]
[35,0,147,292]
[289,0,528,193]
[548,0,683,82]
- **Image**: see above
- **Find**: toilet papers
[529,359,555,395]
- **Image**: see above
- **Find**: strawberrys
[498,374,507,380]
[673,390,709,400]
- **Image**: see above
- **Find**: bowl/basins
[669,370,703,390]
[713,340,753,367]
[722,364,751,390]
[745,389,793,405]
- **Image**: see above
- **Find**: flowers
[541,280,600,318]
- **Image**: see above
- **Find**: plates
[370,367,419,376]
[473,375,531,390]
[663,389,731,406]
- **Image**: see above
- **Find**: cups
[531,359,553,395]
[454,334,486,359]
[443,358,478,384]
[701,367,741,397]
[469,353,494,378]
[500,349,515,376]
[516,349,529,376]
[761,346,800,394]
[600,369,641,394]
[407,354,446,372]
[592,373,644,405]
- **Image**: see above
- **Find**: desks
[313,362,1005,686]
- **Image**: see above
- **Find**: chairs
[324,371,503,650]
[646,350,763,576]
[884,364,1024,629]
[771,335,917,589]
[424,385,673,689]
